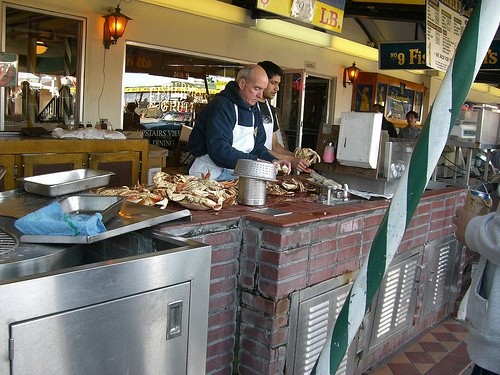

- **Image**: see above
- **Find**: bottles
[323,141,335,163]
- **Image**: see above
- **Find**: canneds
[470,189,491,199]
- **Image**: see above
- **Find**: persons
[453,200,500,375]
[16,81,74,122]
[372,104,398,137]
[188,64,291,181]
[398,111,422,138]
[123,103,140,126]
[255,61,311,172]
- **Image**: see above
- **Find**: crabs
[95,167,239,210]
[294,147,321,165]
[275,164,288,174]
[266,177,316,196]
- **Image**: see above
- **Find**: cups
[237,174,266,205]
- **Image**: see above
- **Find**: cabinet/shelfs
[351,72,425,127]
[0,138,149,191]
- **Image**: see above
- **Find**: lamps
[36,38,49,55]
[101,4,134,49]
[342,62,362,89]
